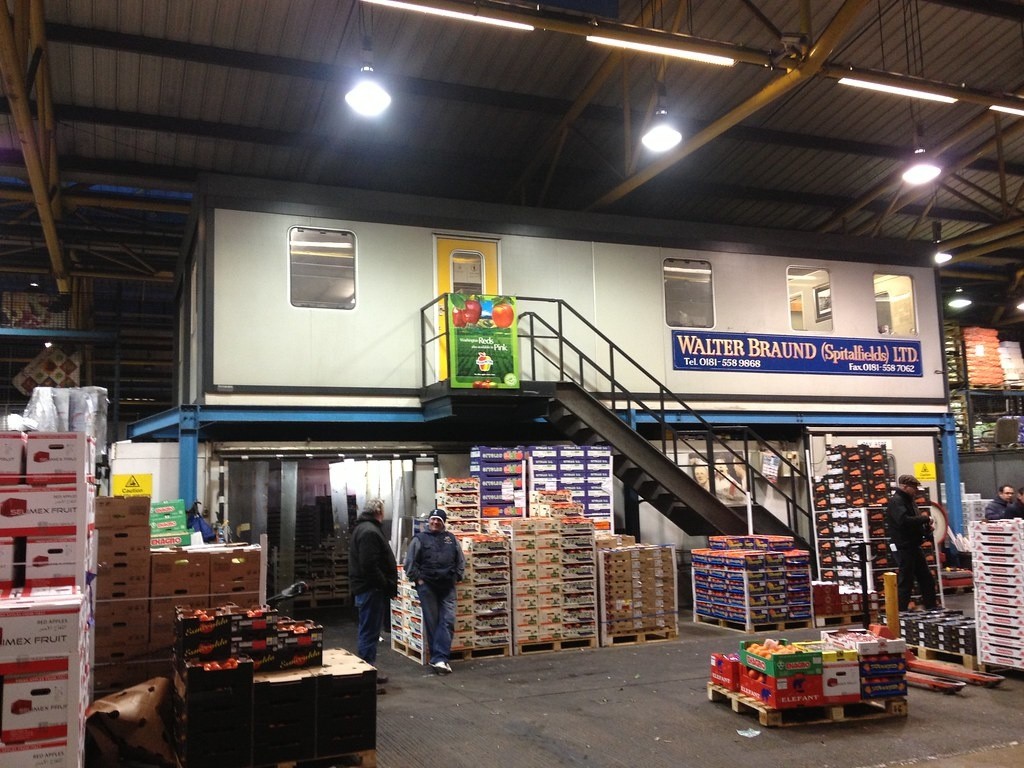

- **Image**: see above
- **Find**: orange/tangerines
[282,625,308,633]
[746,643,802,682]
[195,658,239,672]
[192,609,265,621]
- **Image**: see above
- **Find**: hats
[428,509,446,526]
[899,474,922,486]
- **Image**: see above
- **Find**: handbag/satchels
[187,512,216,542]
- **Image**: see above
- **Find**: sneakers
[445,663,452,673]
[428,660,447,673]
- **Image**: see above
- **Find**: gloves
[1006,502,1017,513]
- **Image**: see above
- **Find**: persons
[985,484,1024,521]
[689,454,746,502]
[404,510,466,674]
[349,498,397,694]
[887,475,939,612]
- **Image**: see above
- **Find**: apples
[452,300,482,327]
[492,303,513,328]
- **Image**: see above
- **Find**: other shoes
[376,673,388,683]
[376,684,386,695]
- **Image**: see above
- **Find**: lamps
[947,261,973,309]
[930,183,953,264]
[640,82,683,150]
[901,0,942,186]
[344,0,392,117]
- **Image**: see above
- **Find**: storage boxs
[0,429,325,768]
[998,341,1024,386]
[389,442,1024,711]
